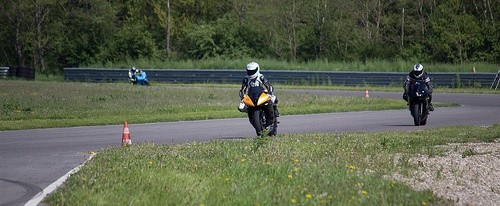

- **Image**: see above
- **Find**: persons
[237,61,280,119]
[129,67,140,83]
[402,64,436,111]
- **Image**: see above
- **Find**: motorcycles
[132,71,151,86]
[407,77,433,127]
[238,86,280,140]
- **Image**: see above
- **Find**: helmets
[246,62,259,79]
[413,63,423,78]
[131,67,136,72]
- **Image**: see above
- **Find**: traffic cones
[121,122,132,146]
[365,89,370,98]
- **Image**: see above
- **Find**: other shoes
[429,102,435,111]
[267,122,274,130]
